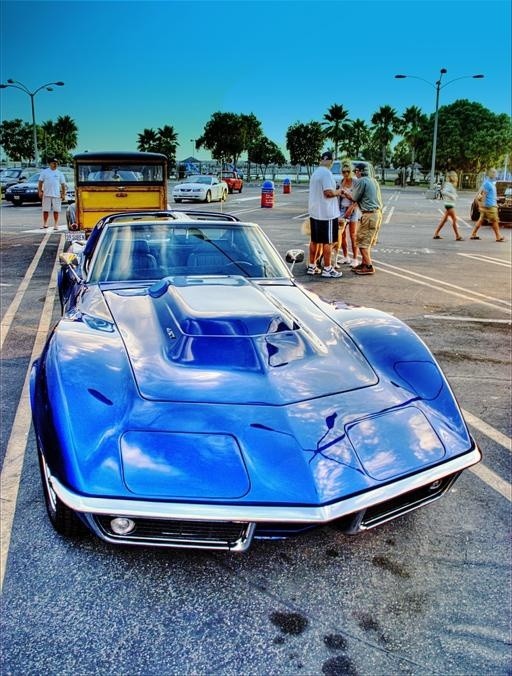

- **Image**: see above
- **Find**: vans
[328,160,375,190]
[73,150,168,240]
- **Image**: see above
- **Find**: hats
[321,152,337,160]
[352,163,368,171]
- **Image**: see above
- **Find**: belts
[362,211,373,213]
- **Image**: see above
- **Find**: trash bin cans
[283,178,291,193]
[261,181,275,208]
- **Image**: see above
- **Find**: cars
[1,167,75,205]
[65,202,77,231]
[456,173,477,188]
[424,171,445,184]
[470,180,511,222]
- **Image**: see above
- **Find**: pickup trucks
[213,171,244,193]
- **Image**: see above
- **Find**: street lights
[233,134,256,172]
[1,78,65,168]
[190,135,200,162]
[393,67,484,199]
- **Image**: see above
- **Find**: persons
[335,159,362,269]
[433,171,463,241]
[306,152,343,278]
[397,165,424,186]
[470,168,508,243]
[39,158,66,230]
[341,161,382,274]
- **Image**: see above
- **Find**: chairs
[100,236,246,282]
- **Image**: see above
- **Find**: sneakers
[307,256,375,278]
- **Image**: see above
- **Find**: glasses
[342,170,350,174]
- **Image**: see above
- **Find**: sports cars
[172,175,228,203]
[28,210,482,556]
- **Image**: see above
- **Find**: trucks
[176,161,244,179]
